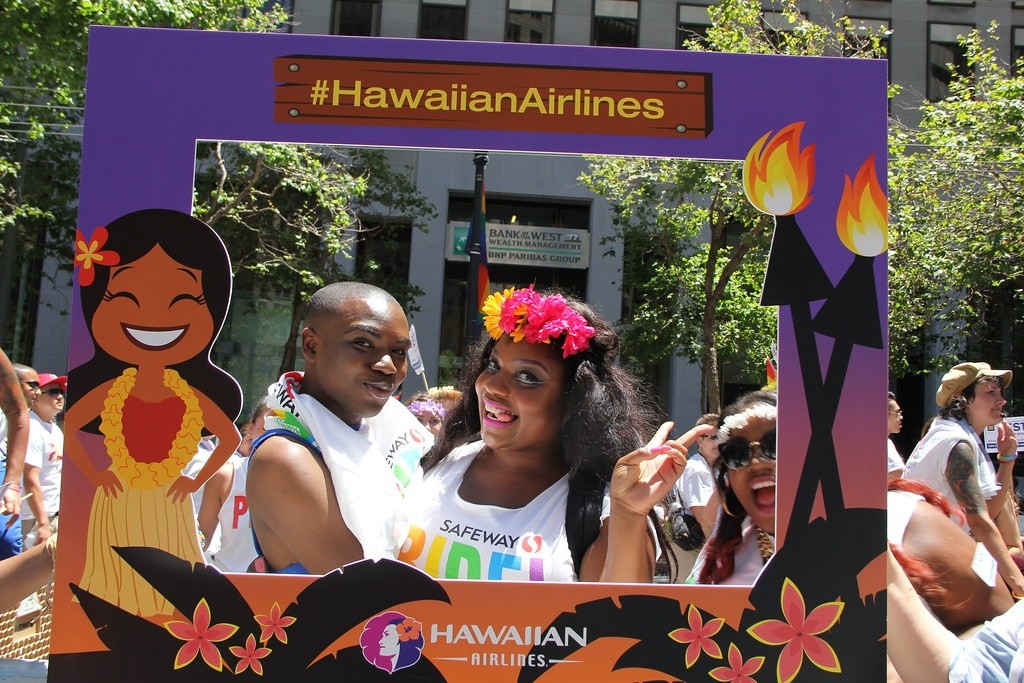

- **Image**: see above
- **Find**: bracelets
[1011,591,1024,599]
[997,450,1018,463]
[45,532,58,564]
[8,487,20,493]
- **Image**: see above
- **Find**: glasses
[20,381,40,389]
[41,388,64,395]
[889,410,901,417]
[699,434,719,440]
[716,428,777,470]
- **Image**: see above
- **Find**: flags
[407,323,425,375]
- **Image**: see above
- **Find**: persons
[418,277,713,584]
[183,281,463,577]
[0,347,68,613]
[672,360,1024,683]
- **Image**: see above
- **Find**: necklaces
[757,527,774,563]
[700,452,712,468]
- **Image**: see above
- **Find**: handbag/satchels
[672,515,706,551]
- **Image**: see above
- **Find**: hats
[936,362,1012,408]
[38,372,68,392]
